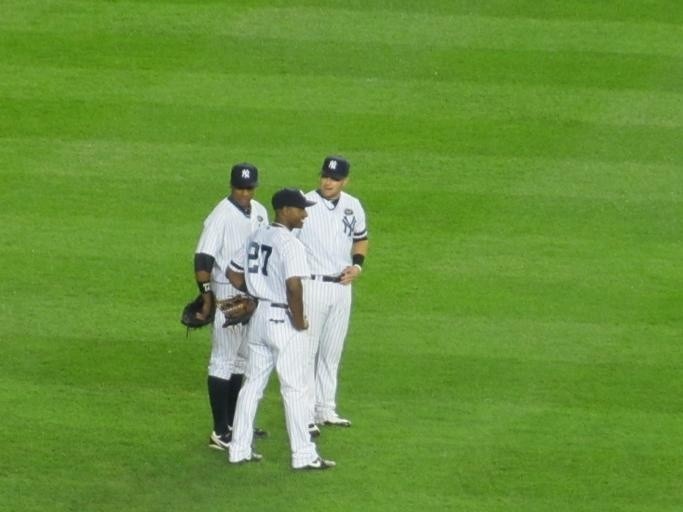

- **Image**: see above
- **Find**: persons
[195,163,268,451]
[291,156,369,435]
[229,189,335,469]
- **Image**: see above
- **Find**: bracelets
[197,281,211,293]
[353,253,364,269]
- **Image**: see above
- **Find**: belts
[270,303,289,310]
[311,274,341,282]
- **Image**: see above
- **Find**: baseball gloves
[180,290,217,335]
[220,296,256,330]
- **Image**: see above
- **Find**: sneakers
[305,458,336,469]
[243,452,263,462]
[323,415,351,427]
[208,424,270,452]
[309,424,321,435]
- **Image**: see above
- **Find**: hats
[271,188,317,208]
[230,162,259,189]
[320,156,350,180]
